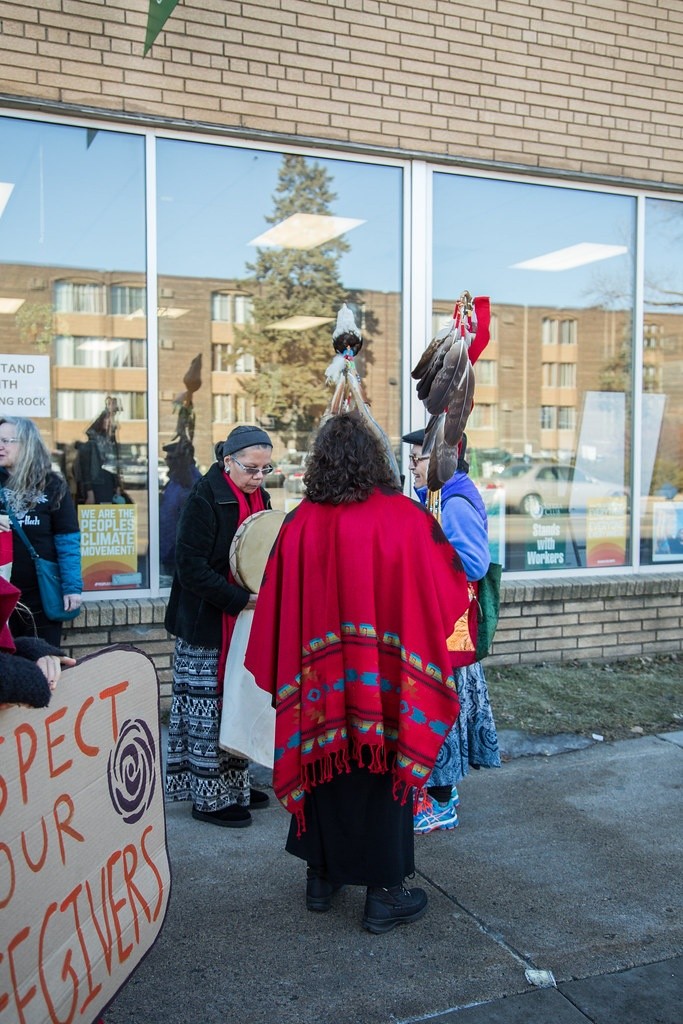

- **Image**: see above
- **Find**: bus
[467,447,513,480]
[513,453,560,480]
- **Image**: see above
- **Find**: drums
[229,509,289,593]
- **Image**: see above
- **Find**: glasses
[232,457,273,475]
[409,454,430,467]
[1,435,23,444]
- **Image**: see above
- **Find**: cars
[117,454,207,488]
[265,451,314,493]
[474,463,631,514]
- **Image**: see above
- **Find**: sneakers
[451,787,461,807]
[363,887,429,934]
[411,800,460,836]
[305,879,343,912]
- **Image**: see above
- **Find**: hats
[402,429,467,460]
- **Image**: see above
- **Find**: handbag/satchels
[476,562,502,661]
[35,558,81,621]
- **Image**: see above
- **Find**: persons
[0,635,77,710]
[1,418,82,649]
[401,428,502,837]
[164,425,272,828]
[159,442,203,584]
[243,413,476,935]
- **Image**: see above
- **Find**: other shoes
[244,788,271,809]
[191,803,252,828]
